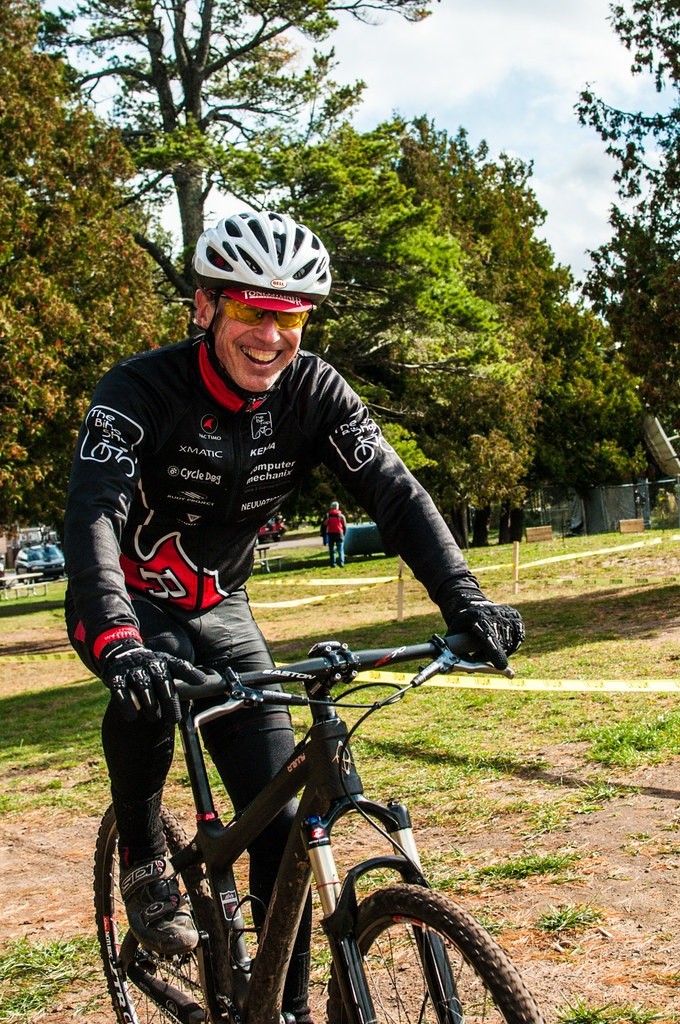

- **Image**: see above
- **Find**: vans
[14,544,65,583]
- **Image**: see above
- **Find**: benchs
[250,545,285,576]
[0,572,53,601]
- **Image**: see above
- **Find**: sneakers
[119,851,200,956]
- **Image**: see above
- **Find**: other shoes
[330,561,345,568]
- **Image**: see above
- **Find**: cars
[0,554,6,589]
[258,513,286,543]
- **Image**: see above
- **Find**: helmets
[191,212,333,311]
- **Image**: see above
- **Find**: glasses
[219,300,310,330]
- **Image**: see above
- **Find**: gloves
[443,587,525,670]
[99,638,205,726]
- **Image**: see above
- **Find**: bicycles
[93,634,547,1024]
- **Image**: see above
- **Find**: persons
[60,212,533,1024]
[323,501,348,568]
